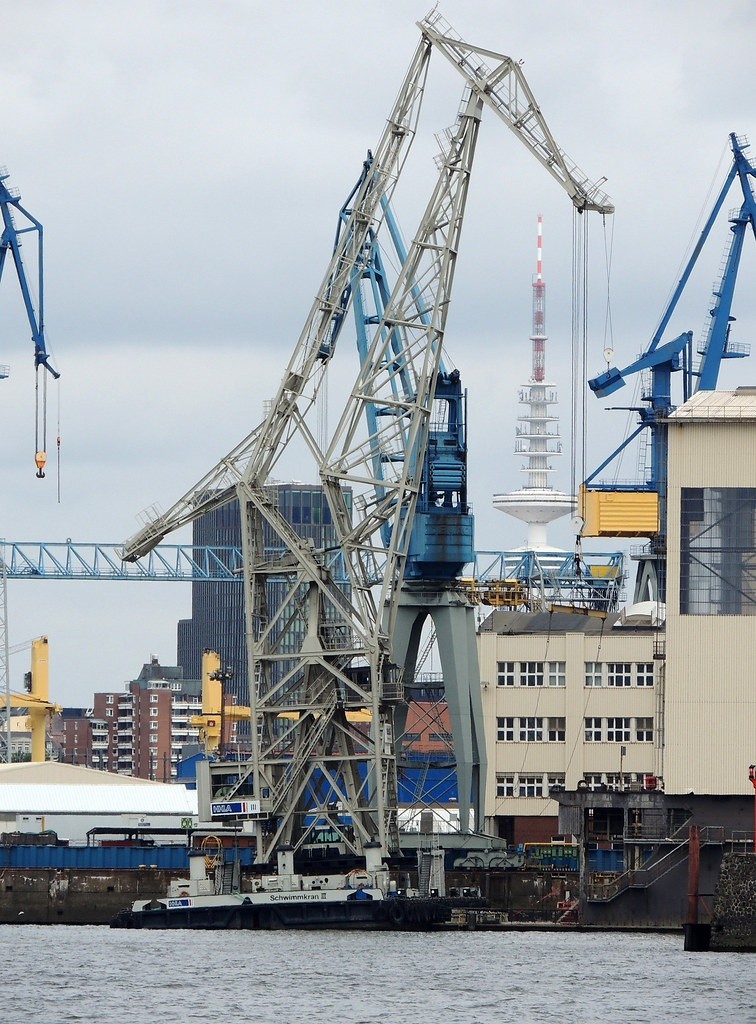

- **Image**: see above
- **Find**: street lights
[206,666,233,759]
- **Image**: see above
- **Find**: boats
[109,836,492,931]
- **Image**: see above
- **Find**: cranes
[120,0,756,859]
